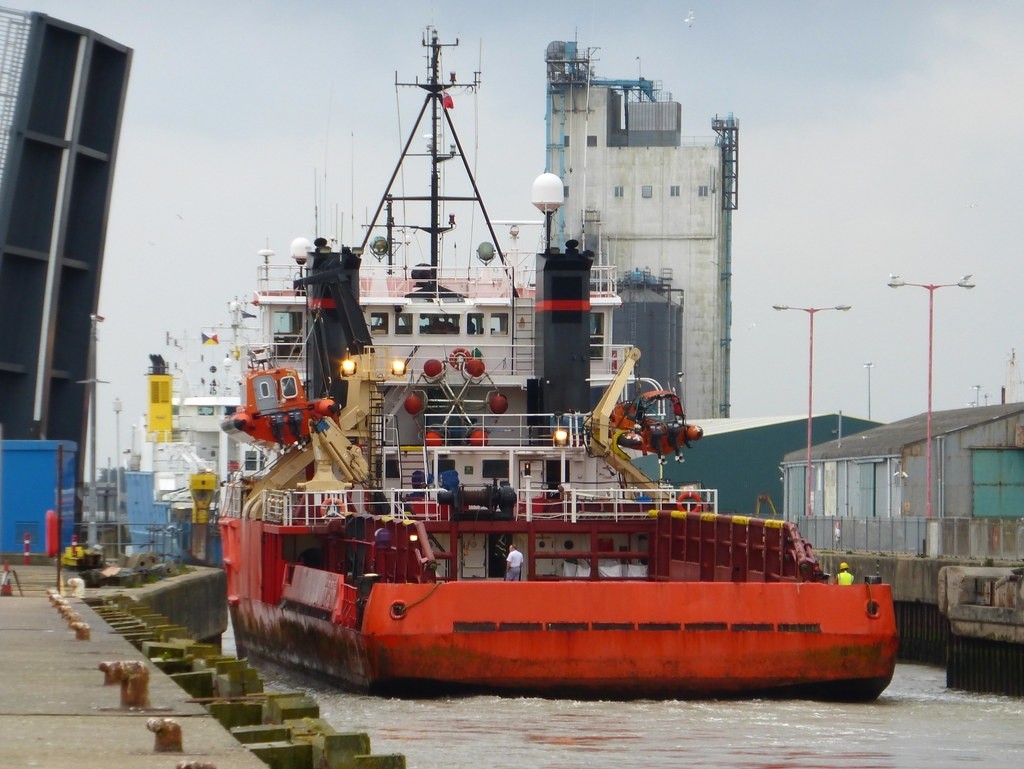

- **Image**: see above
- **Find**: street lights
[773,304,851,516]
[888,274,977,517]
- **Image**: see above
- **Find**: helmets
[840,562,850,570]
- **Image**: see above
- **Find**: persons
[505,544,523,581]
[833,562,854,585]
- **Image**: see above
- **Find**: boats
[213,21,900,704]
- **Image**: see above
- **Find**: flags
[241,310,256,318]
[202,331,219,345]
[444,92,454,109]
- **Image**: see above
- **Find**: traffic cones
[0,561,14,596]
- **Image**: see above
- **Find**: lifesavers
[677,493,701,512]
[320,498,345,522]
[449,348,471,370]
